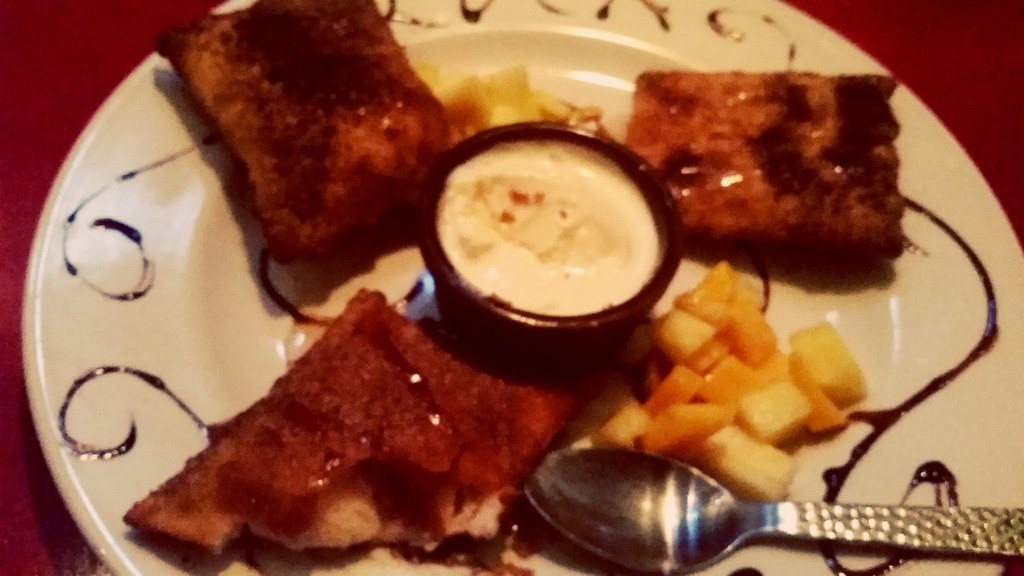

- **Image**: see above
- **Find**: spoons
[522,444,1024,576]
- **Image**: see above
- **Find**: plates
[18,0,1024,576]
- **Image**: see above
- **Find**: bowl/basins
[414,120,686,374]
